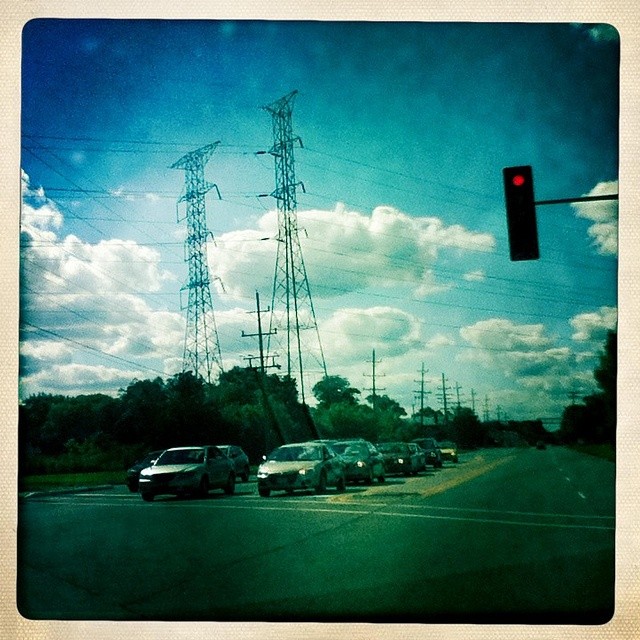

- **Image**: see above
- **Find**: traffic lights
[501,166,541,260]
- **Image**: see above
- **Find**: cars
[214,445,251,483]
[333,442,385,482]
[414,437,442,467]
[257,442,346,495]
[375,442,412,475]
[139,447,236,500]
[313,440,337,454]
[407,444,427,470]
[127,449,165,491]
[439,442,458,462]
